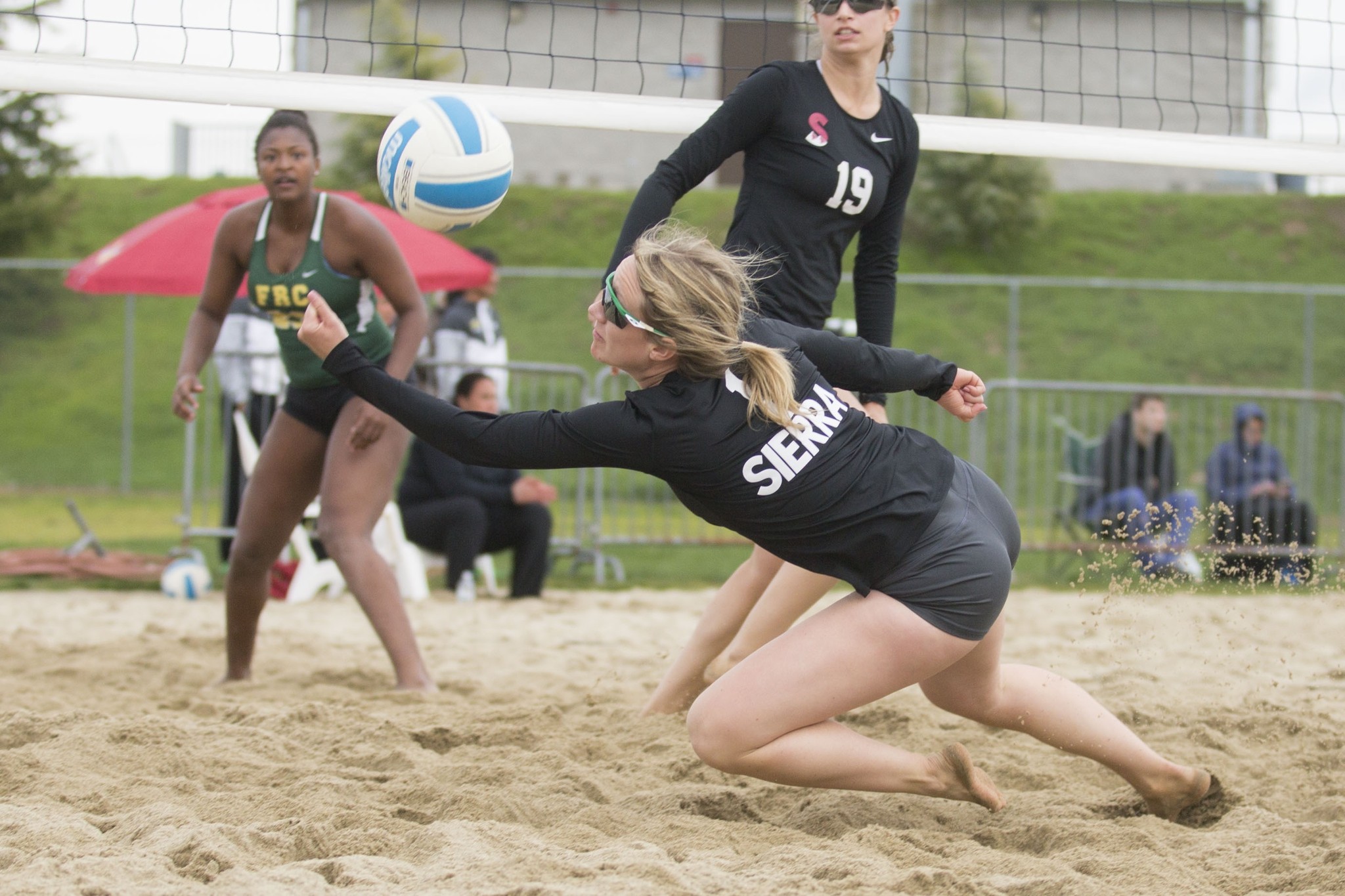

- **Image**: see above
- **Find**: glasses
[809,0,884,14]
[602,271,671,338]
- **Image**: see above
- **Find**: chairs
[1044,412,1151,587]
[231,408,510,607]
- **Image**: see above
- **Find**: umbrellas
[66,182,490,302]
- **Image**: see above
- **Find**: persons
[593,0,921,719]
[430,248,510,423]
[210,286,293,569]
[1205,404,1321,591]
[398,371,557,599]
[170,110,439,695]
[1073,393,1198,580]
[301,221,1219,827]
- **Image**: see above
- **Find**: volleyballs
[374,93,514,232]
[159,557,213,602]
